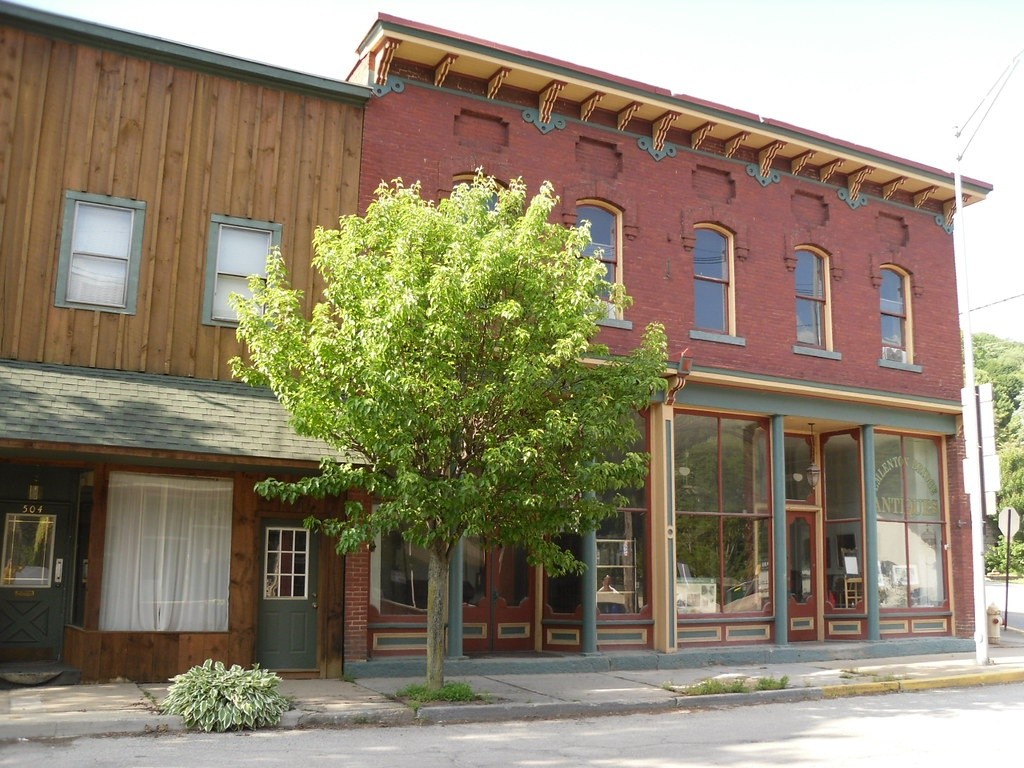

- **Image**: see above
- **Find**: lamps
[806,423,820,491]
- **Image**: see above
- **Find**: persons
[597,573,624,614]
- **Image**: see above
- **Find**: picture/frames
[892,565,918,585]
[841,547,860,579]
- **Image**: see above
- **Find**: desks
[844,574,862,608]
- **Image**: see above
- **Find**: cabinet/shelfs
[597,538,636,614]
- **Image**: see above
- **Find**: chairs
[723,578,762,612]
